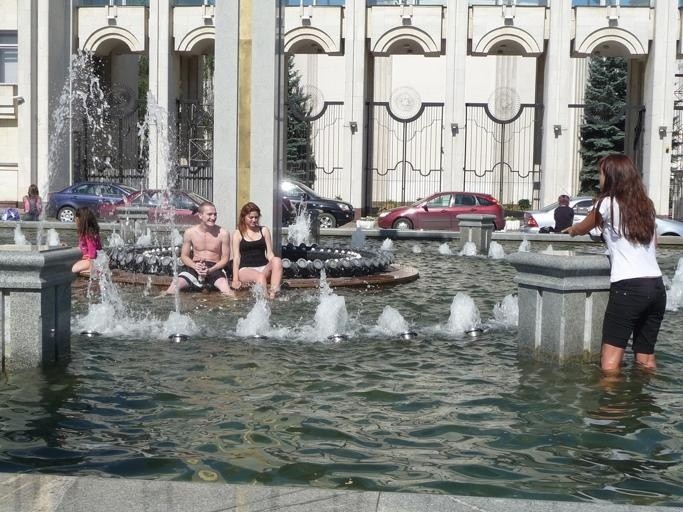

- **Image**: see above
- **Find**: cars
[518,194,683,238]
[281,172,356,230]
[44,177,140,222]
[375,189,507,235]
[92,187,214,226]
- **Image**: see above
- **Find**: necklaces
[244,229,260,240]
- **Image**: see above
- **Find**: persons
[70,206,102,277]
[163,200,235,301]
[584,373,656,442]
[230,201,284,299]
[560,150,667,374]
[552,194,573,233]
[20,184,43,221]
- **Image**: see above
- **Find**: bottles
[196,260,206,284]
